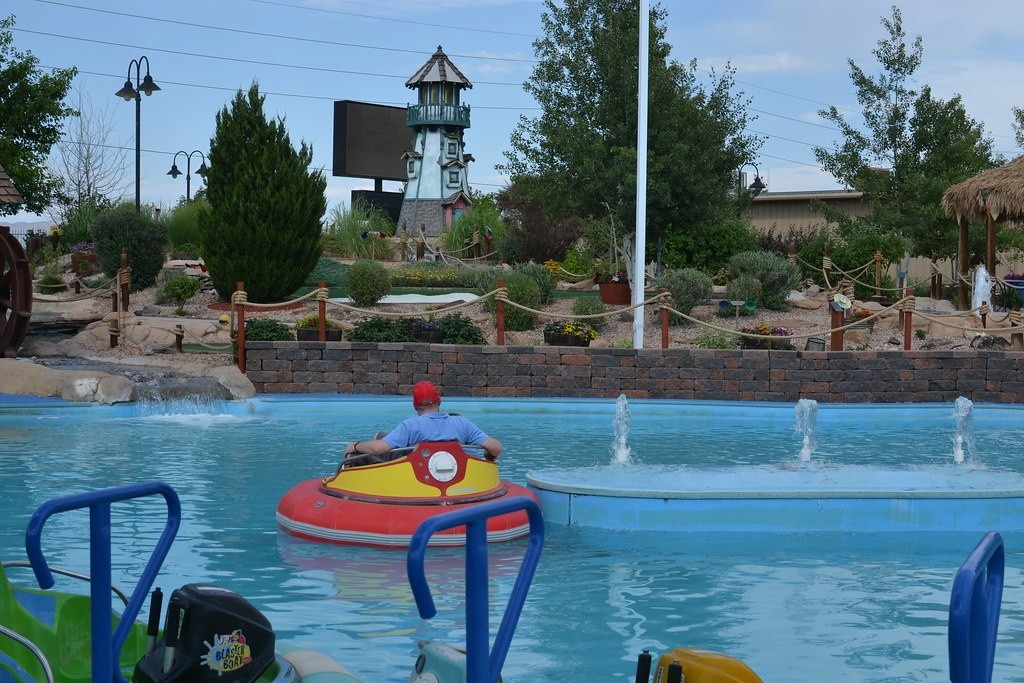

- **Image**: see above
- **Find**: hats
[413,381,440,406]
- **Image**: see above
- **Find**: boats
[274,433,547,552]
[0,483,1002,683]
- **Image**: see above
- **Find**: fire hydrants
[218,313,231,328]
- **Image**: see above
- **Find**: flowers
[592,270,629,285]
[410,306,442,331]
[737,327,796,350]
[847,307,878,322]
[1004,274,1024,280]
[71,242,96,256]
[296,314,334,329]
[543,318,597,343]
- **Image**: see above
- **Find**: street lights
[114,55,162,214]
[165,149,210,205]
[732,160,766,222]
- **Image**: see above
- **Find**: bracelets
[354,441,361,453]
[487,452,496,460]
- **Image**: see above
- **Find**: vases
[598,283,631,305]
[419,331,443,344]
[297,329,342,341]
[544,332,591,347]
[744,339,790,349]
[1007,282,1024,299]
[843,317,873,335]
[70,254,98,272]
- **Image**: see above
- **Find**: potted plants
[231,318,296,366]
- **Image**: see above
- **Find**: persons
[346,381,502,463]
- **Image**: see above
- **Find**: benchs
[324,441,500,497]
[2,562,147,683]
